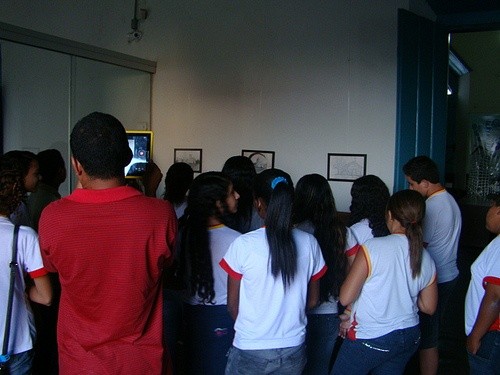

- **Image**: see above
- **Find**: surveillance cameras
[127,31,141,39]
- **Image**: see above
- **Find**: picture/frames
[327,153,366,182]
[241,149,275,174]
[174,148,202,172]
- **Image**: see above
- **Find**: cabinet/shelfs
[0,40,151,200]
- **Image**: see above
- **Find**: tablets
[124,131,153,179]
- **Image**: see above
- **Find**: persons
[0,169,53,375]
[0,149,67,375]
[343,175,390,244]
[38,111,177,375]
[161,161,194,375]
[464,192,500,375]
[166,171,244,375]
[221,155,265,233]
[219,169,327,375]
[292,174,359,375]
[403,156,462,375]
[330,189,438,375]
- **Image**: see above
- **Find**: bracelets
[340,309,351,321]
